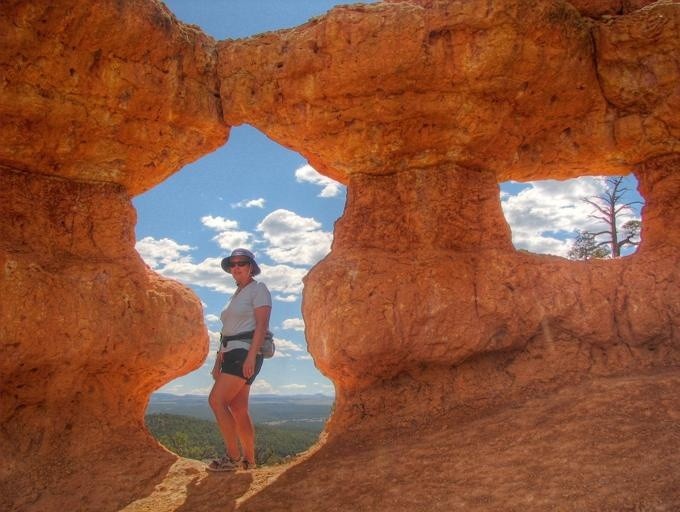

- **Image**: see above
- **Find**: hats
[220,248,261,275]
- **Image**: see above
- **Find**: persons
[206,247,273,472]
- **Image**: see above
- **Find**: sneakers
[242,459,257,470]
[207,452,243,472]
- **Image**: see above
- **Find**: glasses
[227,260,250,268]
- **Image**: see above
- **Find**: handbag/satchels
[243,327,277,358]
